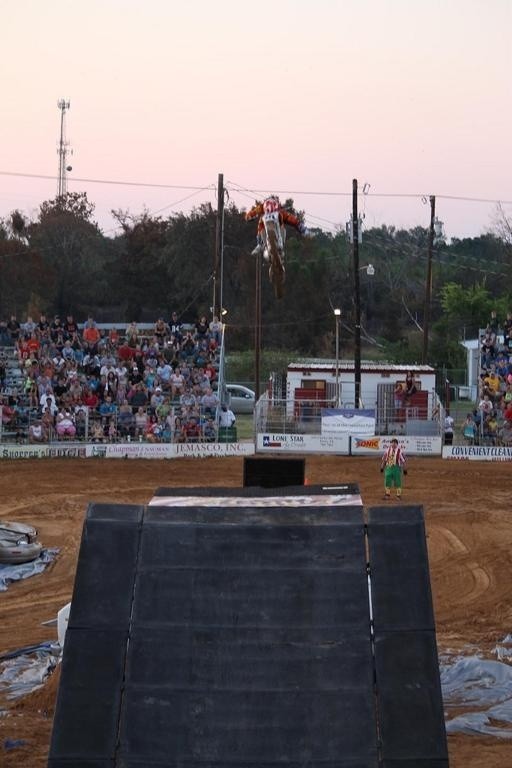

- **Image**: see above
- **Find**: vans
[224,384,256,414]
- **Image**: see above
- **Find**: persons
[380,438,409,502]
[405,370,417,397]
[392,383,404,414]
[245,195,310,257]
[1,310,236,443]
[443,310,512,445]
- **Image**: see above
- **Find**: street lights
[354,264,377,411]
[421,214,446,366]
[209,306,228,327]
[333,308,341,405]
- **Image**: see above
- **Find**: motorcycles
[261,210,288,285]
